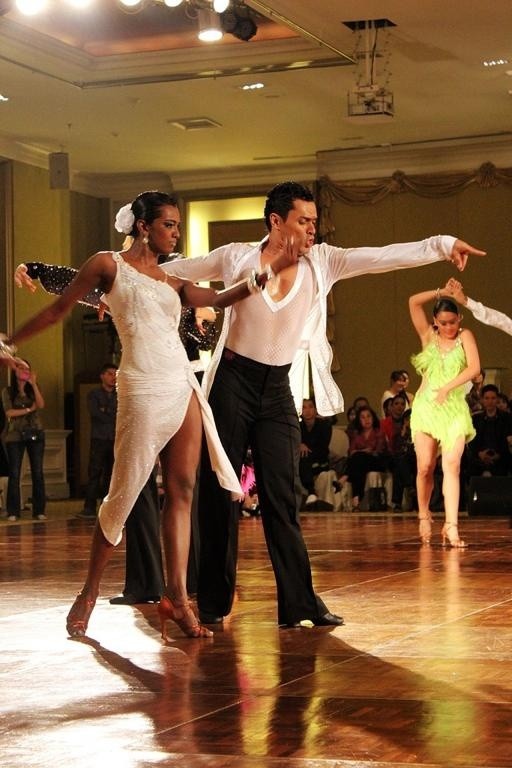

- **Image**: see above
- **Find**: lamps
[194,4,226,44]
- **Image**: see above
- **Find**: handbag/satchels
[22,424,46,441]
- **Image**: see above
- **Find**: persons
[11,232,223,604]
[429,369,511,519]
[1,357,50,520]
[78,361,118,516]
[408,278,482,549]
[447,276,512,338]
[240,452,261,518]
[0,188,303,636]
[96,181,486,628]
[300,369,418,512]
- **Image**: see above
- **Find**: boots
[76,504,97,518]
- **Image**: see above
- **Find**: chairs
[309,427,409,506]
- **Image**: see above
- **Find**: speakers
[347,92,393,119]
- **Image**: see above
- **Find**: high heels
[418,511,434,543]
[157,593,214,639]
[67,593,97,637]
[442,521,469,547]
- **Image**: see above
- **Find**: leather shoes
[110,590,160,605]
[198,607,223,625]
[286,609,343,626]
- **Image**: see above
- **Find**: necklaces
[434,327,461,358]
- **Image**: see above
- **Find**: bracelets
[432,287,441,300]
[265,262,276,281]
[0,332,16,359]
[26,407,32,413]
[248,269,262,293]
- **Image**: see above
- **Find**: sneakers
[8,516,16,521]
[36,514,47,520]
